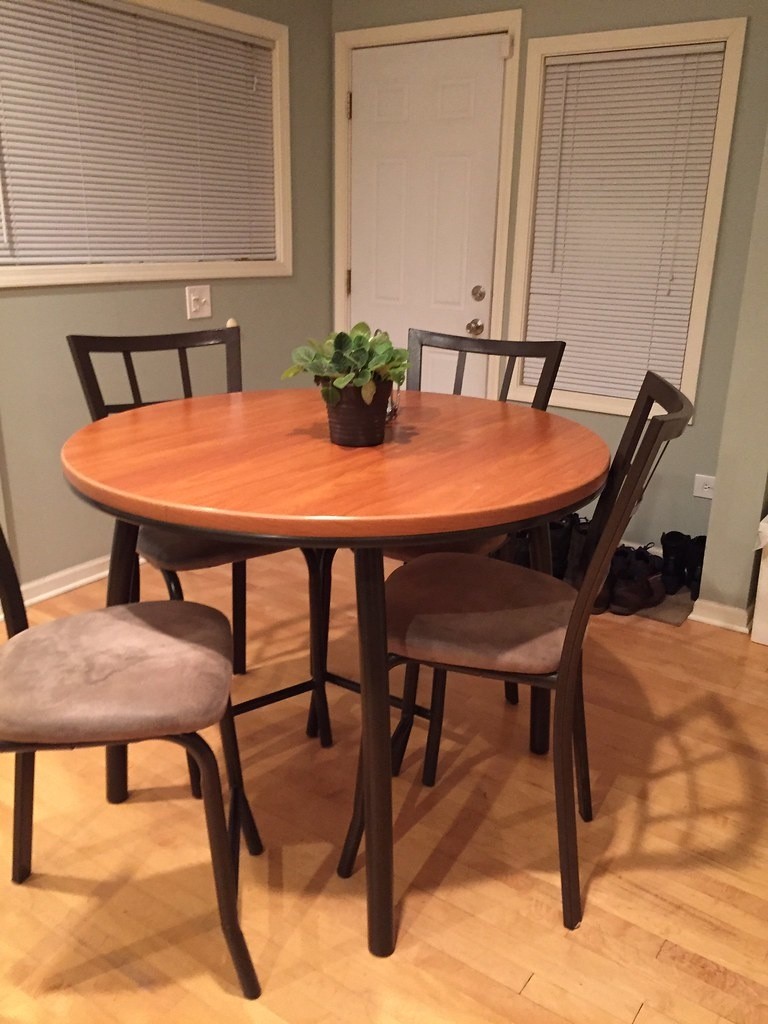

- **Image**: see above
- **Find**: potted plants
[281,322,408,446]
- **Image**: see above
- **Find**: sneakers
[514,512,664,616]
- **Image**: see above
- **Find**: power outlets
[691,474,717,500]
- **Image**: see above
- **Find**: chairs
[65,326,330,804]
[303,325,568,789]
[336,368,697,957]
[0,522,267,1002]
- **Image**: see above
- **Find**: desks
[61,388,614,956]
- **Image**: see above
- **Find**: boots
[660,530,707,600]
